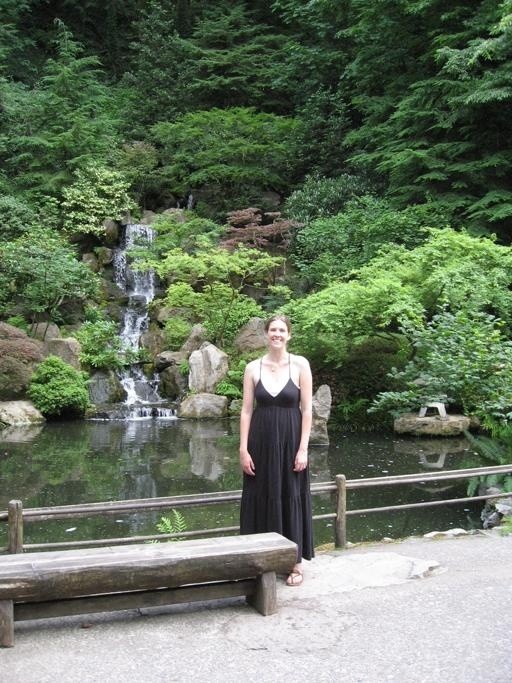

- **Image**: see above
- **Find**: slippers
[286,569,304,586]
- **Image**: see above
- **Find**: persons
[239,316,313,587]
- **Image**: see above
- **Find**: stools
[419,401,446,418]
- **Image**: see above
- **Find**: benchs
[0,531,299,647]
